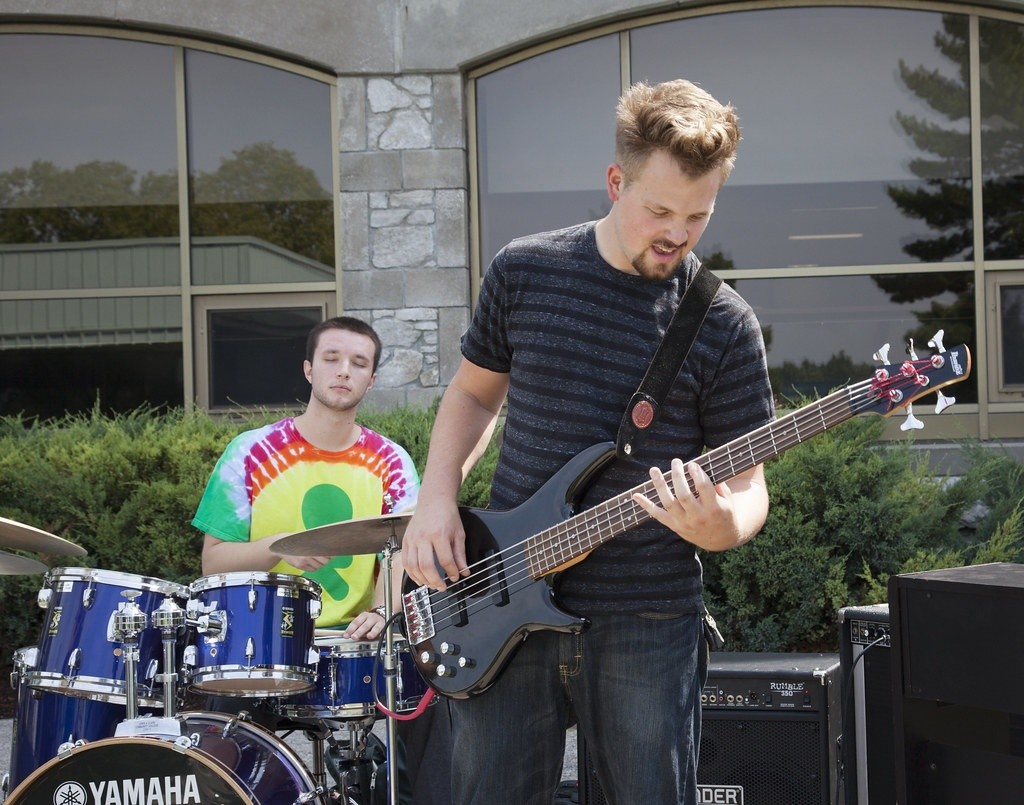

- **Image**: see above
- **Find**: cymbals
[0,517,88,558]
[0,550,48,575]
[270,514,412,557]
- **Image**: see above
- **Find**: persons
[401,73,777,805]
[191,316,422,641]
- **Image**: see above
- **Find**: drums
[2,645,164,797]
[184,571,322,698]
[28,566,191,708]
[273,636,439,719]
[3,710,326,805]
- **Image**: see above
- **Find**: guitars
[400,328,971,699]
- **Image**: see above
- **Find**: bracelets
[369,605,397,627]
